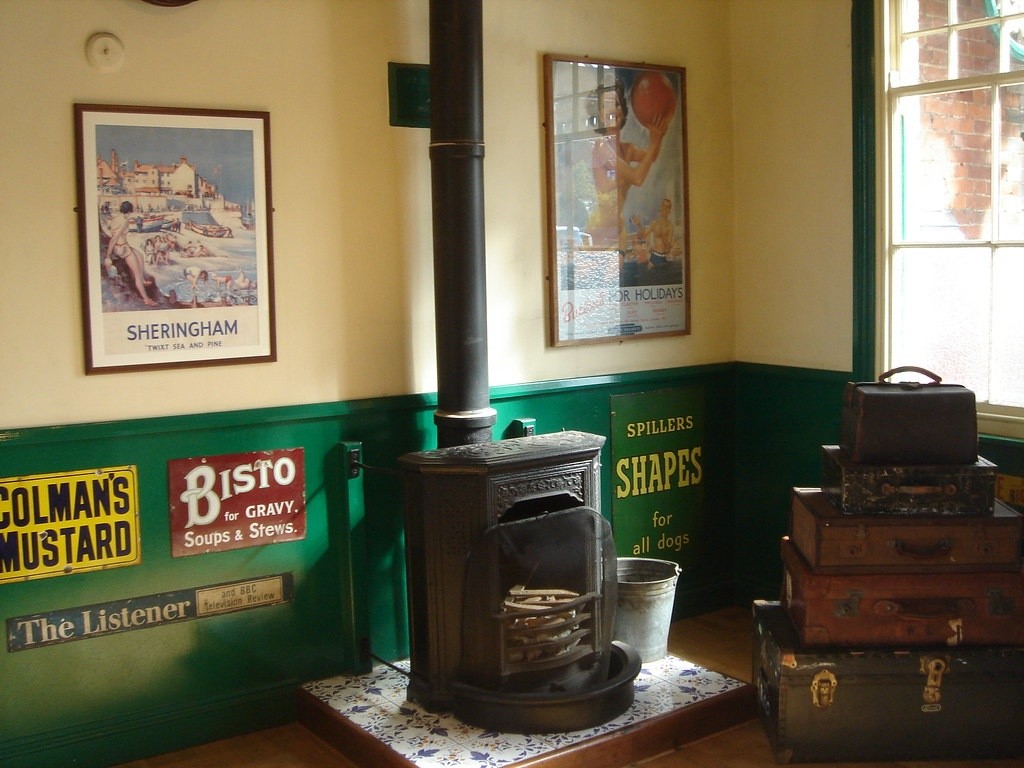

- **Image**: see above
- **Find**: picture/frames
[73,103,277,375]
[543,54,691,347]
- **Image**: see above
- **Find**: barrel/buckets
[613,556,683,664]
